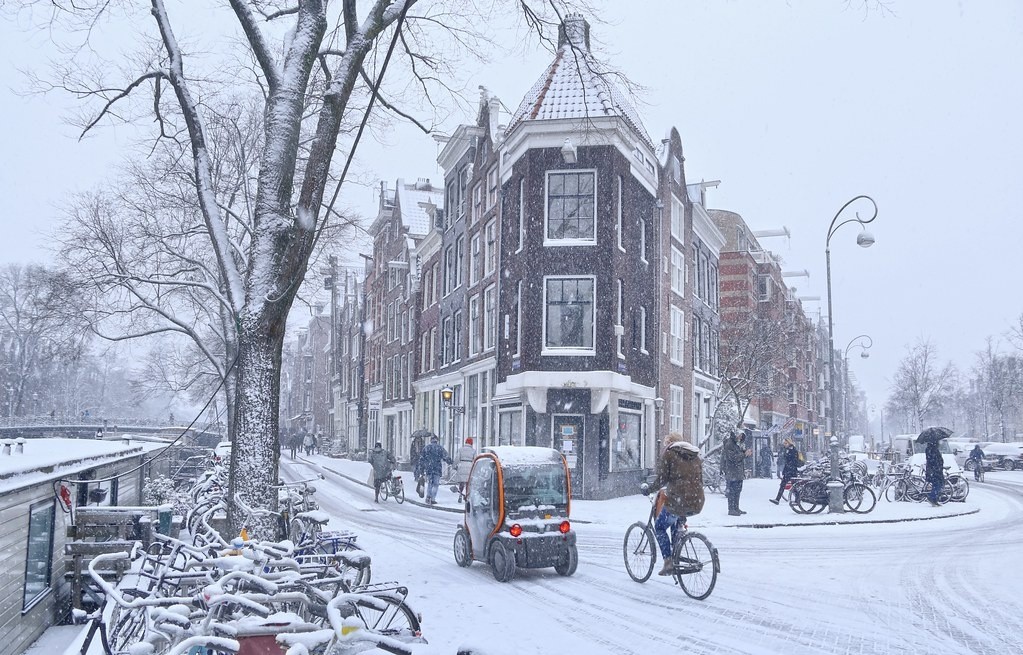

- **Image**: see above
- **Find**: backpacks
[796,449,807,468]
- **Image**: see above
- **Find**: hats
[466,438,472,445]
[430,436,438,442]
[664,432,683,447]
[375,442,381,448]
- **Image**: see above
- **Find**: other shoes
[426,498,429,503]
[932,502,941,507]
[927,497,942,507]
[431,501,437,504]
[769,499,779,505]
[728,510,746,516]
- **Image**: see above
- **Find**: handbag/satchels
[367,468,374,486]
[416,476,424,498]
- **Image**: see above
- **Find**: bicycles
[781,449,876,514]
[623,483,721,601]
[971,456,986,483]
[88,455,478,655]
[872,460,969,504]
[379,474,405,504]
[701,457,728,493]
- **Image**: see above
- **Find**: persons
[769,437,801,505]
[476,462,533,508]
[419,434,453,505]
[719,428,753,516]
[279,426,323,460]
[452,438,476,503]
[970,443,986,480]
[760,443,786,479]
[925,440,945,508]
[367,442,396,503]
[170,412,175,426]
[410,437,426,482]
[877,442,880,452]
[51,410,55,424]
[641,432,705,575]
[80,410,89,424]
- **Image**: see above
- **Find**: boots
[458,495,462,502]
[659,557,673,575]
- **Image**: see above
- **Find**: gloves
[641,489,650,496]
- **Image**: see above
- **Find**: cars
[986,443,1023,471]
[895,435,961,484]
[211,442,232,465]
[950,442,1000,472]
[450,446,578,582]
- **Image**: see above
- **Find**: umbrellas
[915,427,954,444]
[410,429,431,446]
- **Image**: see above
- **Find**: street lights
[829,196,877,514]
[844,335,872,451]
[9,389,13,417]
[866,403,876,440]
[33,394,37,418]
